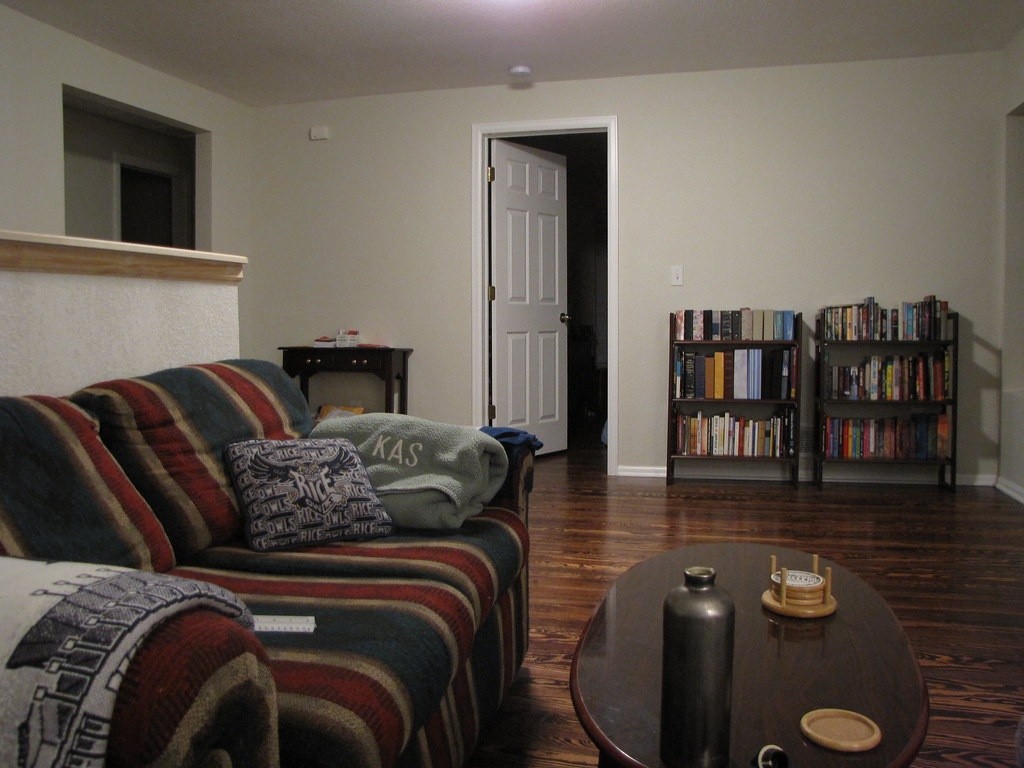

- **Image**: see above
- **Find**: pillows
[224,438,394,552]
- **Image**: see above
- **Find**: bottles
[660,566,736,768]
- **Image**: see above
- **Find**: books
[674,308,798,457]
[820,295,952,462]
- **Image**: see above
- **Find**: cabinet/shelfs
[666,312,803,489]
[815,312,959,489]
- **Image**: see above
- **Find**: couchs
[0,359,543,768]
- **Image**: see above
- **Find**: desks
[277,346,414,414]
[570,542,930,768]
[0,229,248,398]
